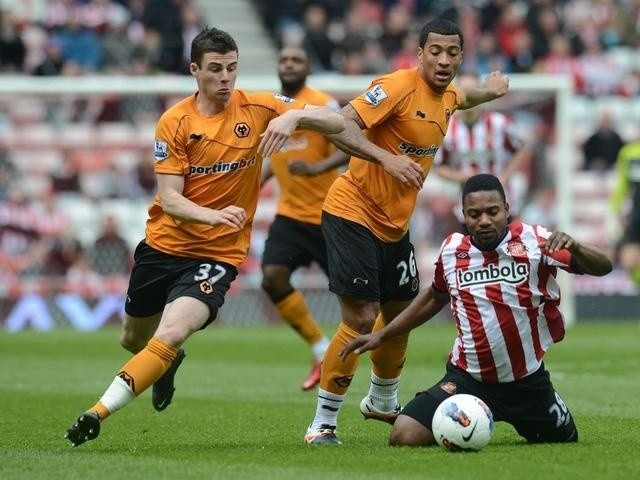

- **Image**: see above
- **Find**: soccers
[431,394,494,451]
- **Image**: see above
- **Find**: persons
[62,28,347,451]
[302,16,513,449]
[0,0,640,301]
[337,173,616,448]
[258,45,353,395]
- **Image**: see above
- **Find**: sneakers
[357,394,407,424]
[299,360,325,392]
[303,425,344,447]
[61,410,103,451]
[150,345,187,414]
